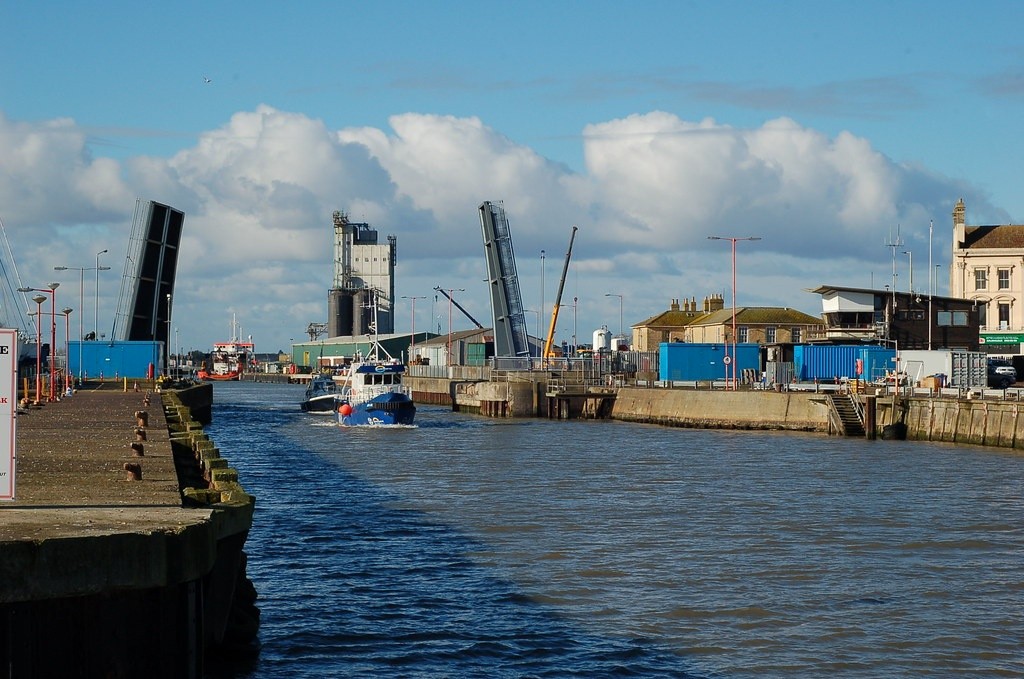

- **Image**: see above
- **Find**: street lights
[175,327,182,380]
[928,219,935,350]
[397,295,426,366]
[31,292,48,406]
[26,306,72,396]
[94,250,109,342]
[933,264,942,296]
[525,309,539,357]
[705,236,763,394]
[902,251,914,306]
[604,293,623,346]
[166,294,173,376]
[53,265,111,387]
[859,336,899,396]
[14,282,58,404]
[436,287,465,367]
[539,249,546,371]
[558,295,579,357]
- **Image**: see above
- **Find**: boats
[295,290,419,428]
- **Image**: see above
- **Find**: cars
[987,358,1019,389]
